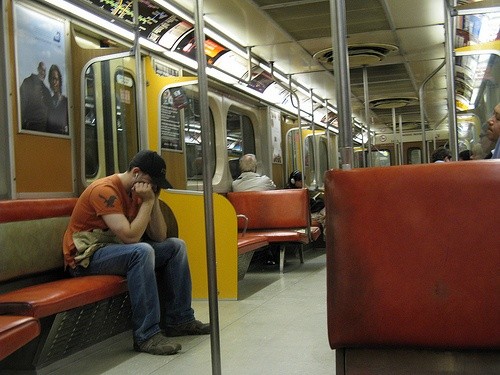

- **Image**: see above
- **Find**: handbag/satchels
[311,200,324,213]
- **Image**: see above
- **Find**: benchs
[0,198,137,371]
[0,315,41,375]
[227,188,322,273]
[237,215,268,282]
[326,159,500,375]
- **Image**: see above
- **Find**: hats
[432,149,452,161]
[130,151,173,189]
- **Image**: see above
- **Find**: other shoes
[272,260,286,265]
[264,260,272,265]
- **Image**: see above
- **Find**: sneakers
[133,332,182,354]
[165,320,211,337]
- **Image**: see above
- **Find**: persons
[485,103,500,159]
[63,151,212,354]
[190,156,204,180]
[479,119,496,159]
[431,148,452,163]
[47,64,70,136]
[288,170,326,247]
[232,154,288,265]
[20,61,50,132]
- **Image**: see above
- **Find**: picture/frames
[12,0,72,139]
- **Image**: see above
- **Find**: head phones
[291,171,299,184]
